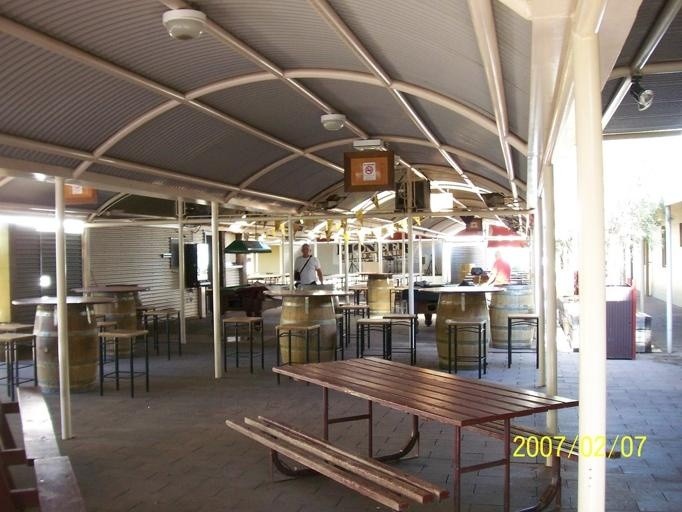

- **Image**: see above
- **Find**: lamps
[163,7,209,40]
[224,223,272,255]
[629,68,655,113]
[320,113,345,131]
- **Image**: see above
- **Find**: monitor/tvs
[183,241,213,287]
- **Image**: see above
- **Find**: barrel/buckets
[367,277,396,319]
[31,304,100,393]
[82,284,143,357]
[435,292,491,370]
[279,282,341,383]
[489,284,536,349]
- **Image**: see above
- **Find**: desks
[270,354,579,511]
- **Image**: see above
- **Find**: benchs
[226,412,450,512]
[12,452,86,512]
[461,422,621,507]
[0,384,61,465]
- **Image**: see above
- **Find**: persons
[479,251,510,309]
[293,243,324,288]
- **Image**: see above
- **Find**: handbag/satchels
[293,271,300,281]
